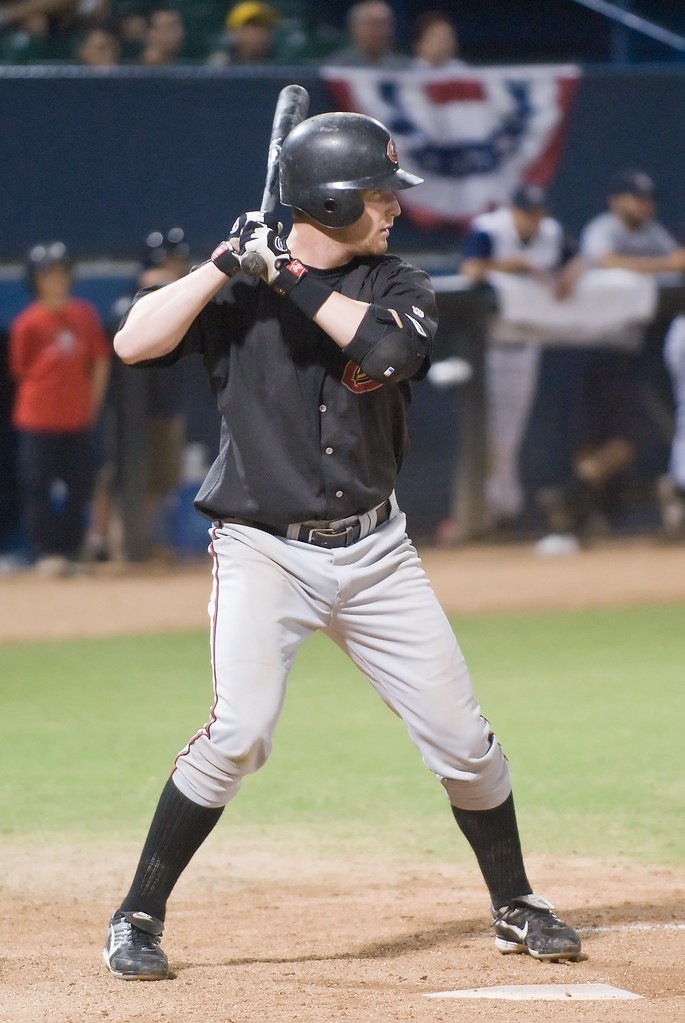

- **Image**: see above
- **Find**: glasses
[146,226,184,248]
[31,241,67,261]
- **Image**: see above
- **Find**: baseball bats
[240,84,312,277]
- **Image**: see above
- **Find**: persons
[102,227,196,562]
[329,0,466,68]
[458,168,685,537]
[100,112,581,982]
[80,1,272,68]
[10,240,106,566]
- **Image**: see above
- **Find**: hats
[515,185,544,209]
[614,167,658,195]
[225,0,276,33]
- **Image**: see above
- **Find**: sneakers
[491,893,582,959]
[102,904,168,982]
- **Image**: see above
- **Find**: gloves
[231,220,335,321]
[212,211,285,278]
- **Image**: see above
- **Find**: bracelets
[288,276,334,317]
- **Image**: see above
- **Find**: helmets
[278,112,424,229]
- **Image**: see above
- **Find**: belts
[222,502,391,550]
